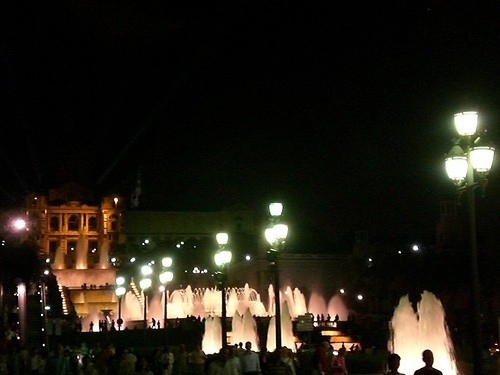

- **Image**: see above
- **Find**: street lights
[263,199,290,348]
[140,263,153,328]
[158,255,173,328]
[114,274,126,331]
[443,95,498,375]
[213,229,233,345]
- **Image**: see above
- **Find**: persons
[0,311,360,375]
[413,348,443,375]
[383,353,405,375]
